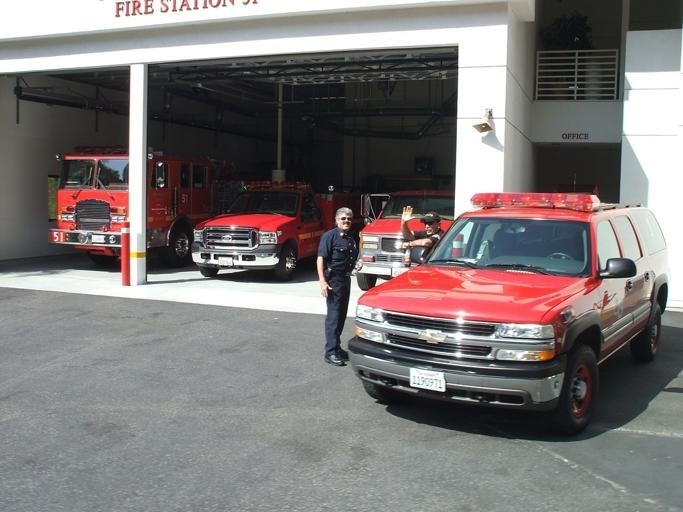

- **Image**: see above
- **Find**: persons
[399,206,444,268]
[316,205,363,366]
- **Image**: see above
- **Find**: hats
[420,212,441,223]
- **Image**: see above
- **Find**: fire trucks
[352,188,454,290]
[46,143,240,269]
[190,177,352,283]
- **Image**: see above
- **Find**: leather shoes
[326,349,349,367]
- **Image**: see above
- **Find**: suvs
[342,188,670,435]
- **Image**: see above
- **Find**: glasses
[339,217,352,221]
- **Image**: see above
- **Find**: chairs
[483,227,519,258]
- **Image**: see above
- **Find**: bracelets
[407,240,412,246]
